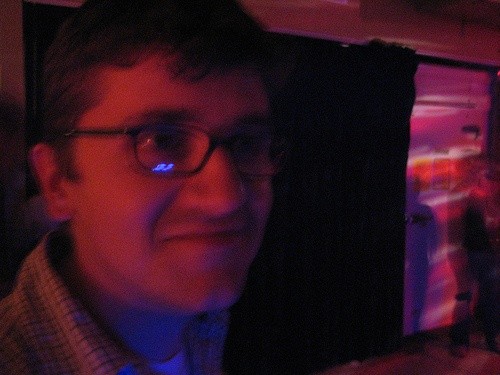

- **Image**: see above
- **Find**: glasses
[56,121,291,183]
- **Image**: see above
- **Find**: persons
[0,0,285,375]
